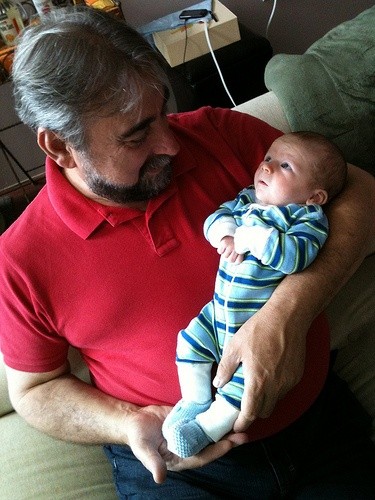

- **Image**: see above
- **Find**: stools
[136,17,274,107]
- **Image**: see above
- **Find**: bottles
[33,0,54,18]
[0,0,25,47]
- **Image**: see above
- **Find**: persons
[162,131,347,457]
[0,6,375,500]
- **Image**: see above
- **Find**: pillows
[263,3,375,177]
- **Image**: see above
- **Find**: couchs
[0,90,375,500]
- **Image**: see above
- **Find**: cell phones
[179,9,207,19]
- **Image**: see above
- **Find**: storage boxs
[147,0,241,67]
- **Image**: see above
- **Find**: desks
[0,54,197,204]
